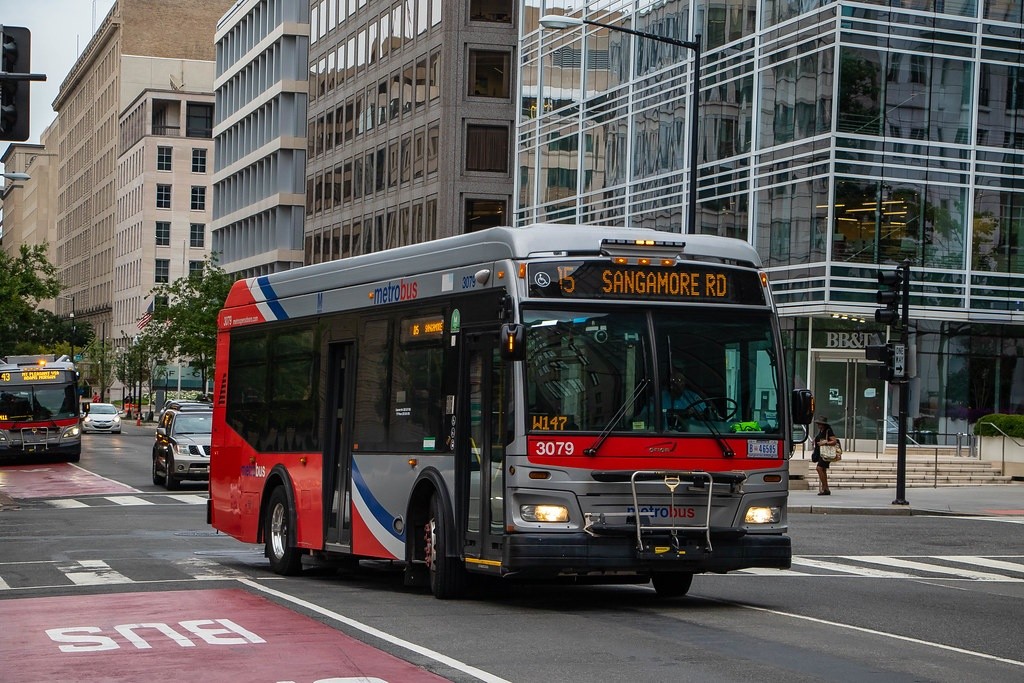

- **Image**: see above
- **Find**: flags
[137,301,154,330]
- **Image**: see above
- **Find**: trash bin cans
[145,411,153,422]
[912,414,938,444]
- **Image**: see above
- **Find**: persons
[124,393,133,403]
[811,416,837,495]
[92,392,100,403]
[641,367,706,423]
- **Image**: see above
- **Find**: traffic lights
[865,343,893,380]
[875,270,902,325]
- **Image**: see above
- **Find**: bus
[0,354,90,467]
[208,223,816,599]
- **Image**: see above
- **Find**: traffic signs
[894,345,905,377]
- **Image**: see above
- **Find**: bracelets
[824,442,827,446]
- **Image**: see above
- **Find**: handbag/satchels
[819,429,842,462]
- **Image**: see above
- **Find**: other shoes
[817,490,831,495]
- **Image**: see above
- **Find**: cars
[80,402,124,434]
[152,399,213,490]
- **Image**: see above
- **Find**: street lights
[69,297,76,362]
[539,14,702,233]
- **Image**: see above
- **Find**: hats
[813,415,829,423]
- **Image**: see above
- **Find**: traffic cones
[137,412,140,426]
